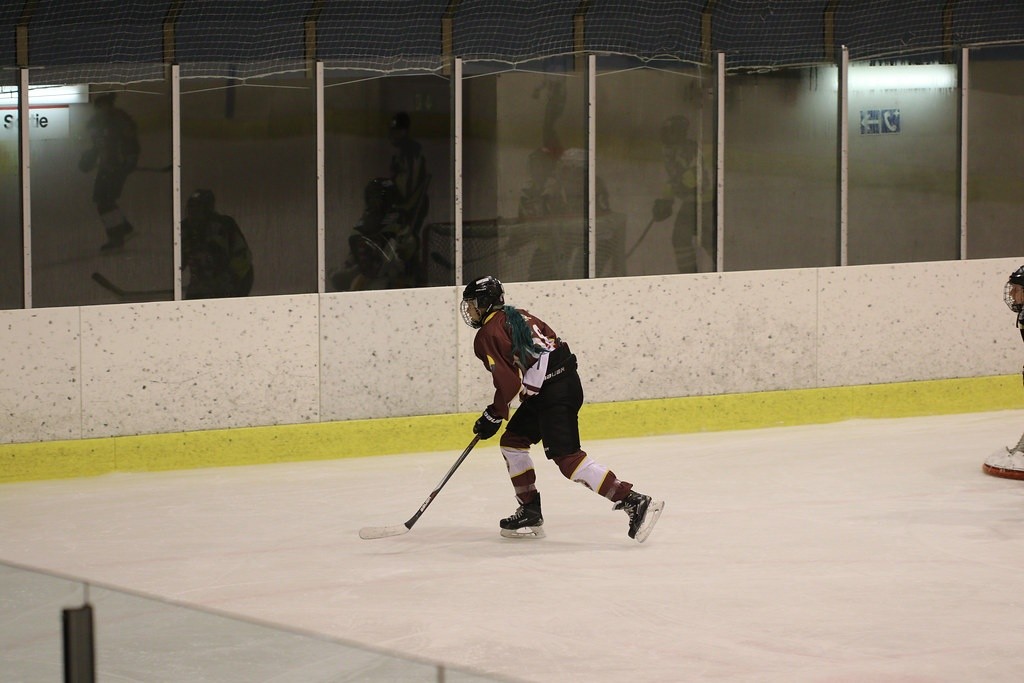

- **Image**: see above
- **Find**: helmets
[389,111,408,129]
[663,114,689,128]
[463,275,505,318]
[187,189,213,205]
[90,90,116,107]
[1007,265,1024,285]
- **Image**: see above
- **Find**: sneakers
[500,492,545,538]
[611,489,665,543]
[102,236,127,255]
[120,225,139,241]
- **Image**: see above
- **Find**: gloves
[473,405,503,440]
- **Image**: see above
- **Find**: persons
[462,276,665,543]
[79,91,716,296]
[1005,267,1024,374]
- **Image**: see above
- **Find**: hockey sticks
[358,433,481,540]
